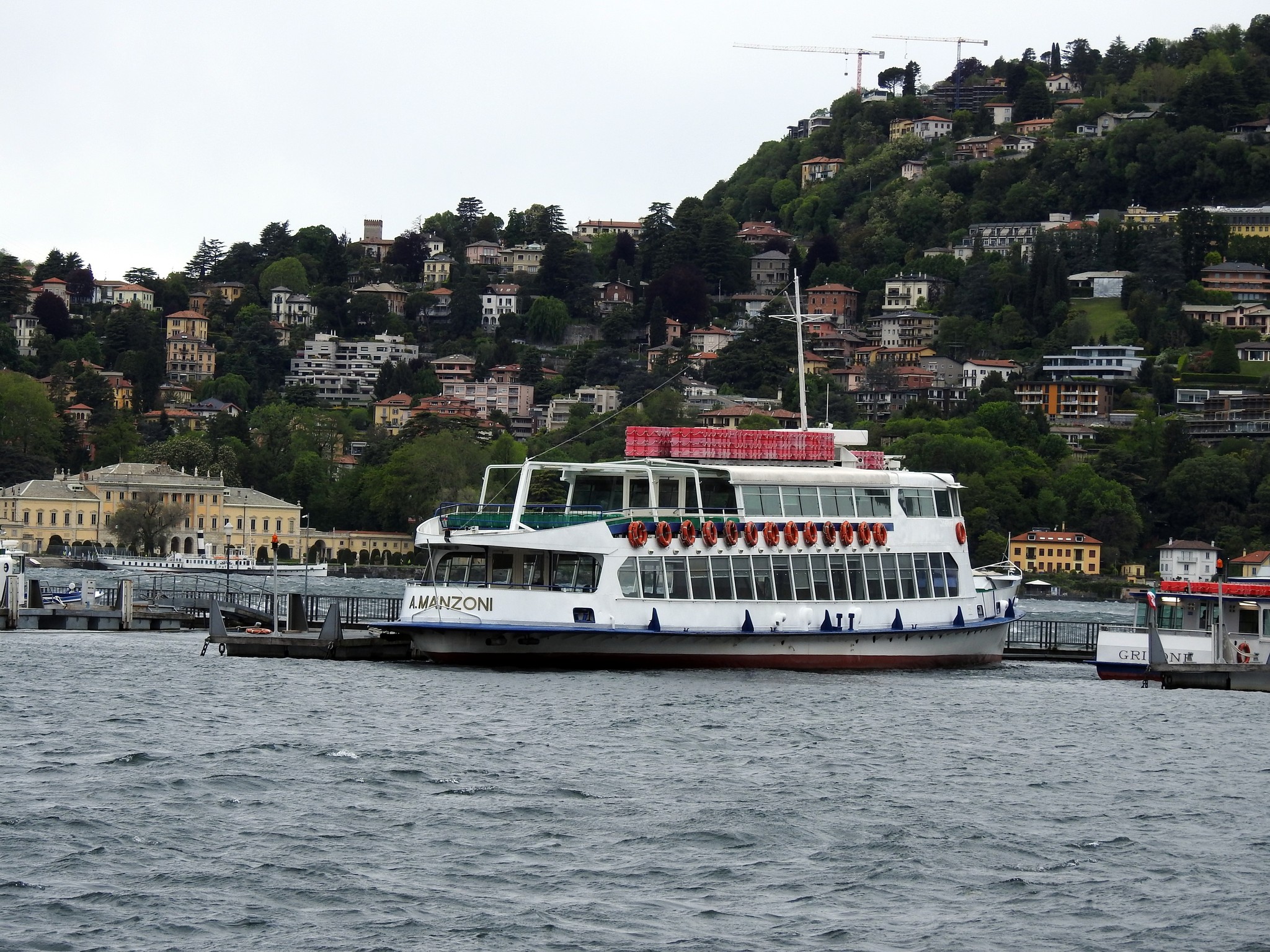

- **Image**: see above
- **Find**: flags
[1147,588,1157,610]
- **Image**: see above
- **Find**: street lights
[223,523,234,603]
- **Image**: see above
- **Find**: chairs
[447,512,740,538]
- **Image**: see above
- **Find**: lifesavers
[702,520,717,547]
[956,522,966,544]
[872,523,887,546]
[822,521,836,547]
[839,521,853,547]
[744,521,758,547]
[1237,643,1250,663]
[656,521,672,548]
[724,520,738,547]
[764,522,780,546]
[803,521,818,547]
[680,520,696,547]
[784,521,798,546]
[857,522,871,546]
[246,628,272,635]
[628,521,647,548]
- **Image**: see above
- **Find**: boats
[1083,557,1270,693]
[0,529,105,610]
[386,267,1024,669]
[93,522,329,578]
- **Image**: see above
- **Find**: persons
[400,559,410,567]
[316,551,319,560]
[169,550,171,555]
[112,553,116,560]
[1199,610,1206,629]
[260,558,266,564]
[104,553,107,559]
[145,553,148,560]
[356,552,359,561]
[63,548,97,560]
[384,552,387,560]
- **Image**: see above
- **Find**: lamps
[1161,597,1182,602]
